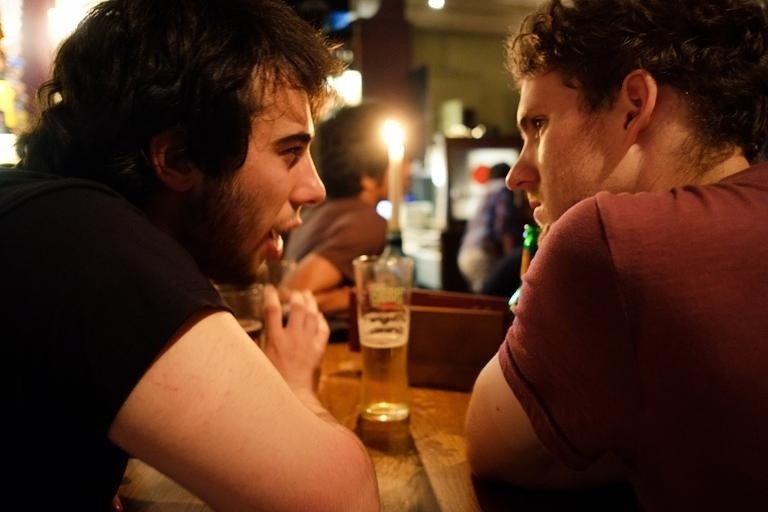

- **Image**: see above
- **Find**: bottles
[509,224,540,340]
[374,228,416,282]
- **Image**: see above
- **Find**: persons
[1,0,383,512]
[457,164,528,294]
[278,131,393,313]
[464,0,768,510]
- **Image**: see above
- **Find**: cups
[354,255,414,425]
